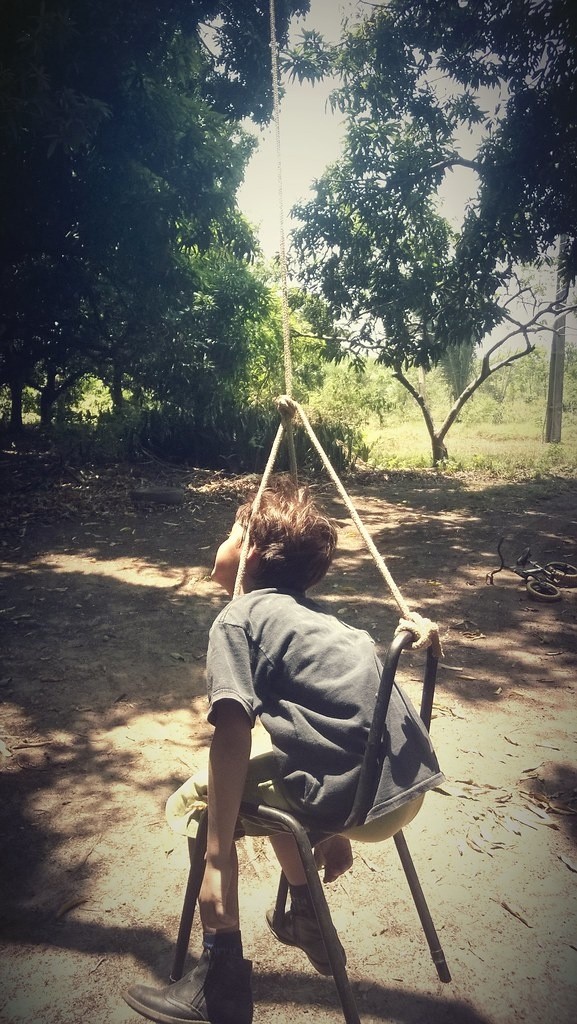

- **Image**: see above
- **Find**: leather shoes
[266,906,346,974]
[121,951,253,1024]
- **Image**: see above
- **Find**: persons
[123,490,445,1024]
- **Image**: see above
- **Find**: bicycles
[485,536,577,601]
